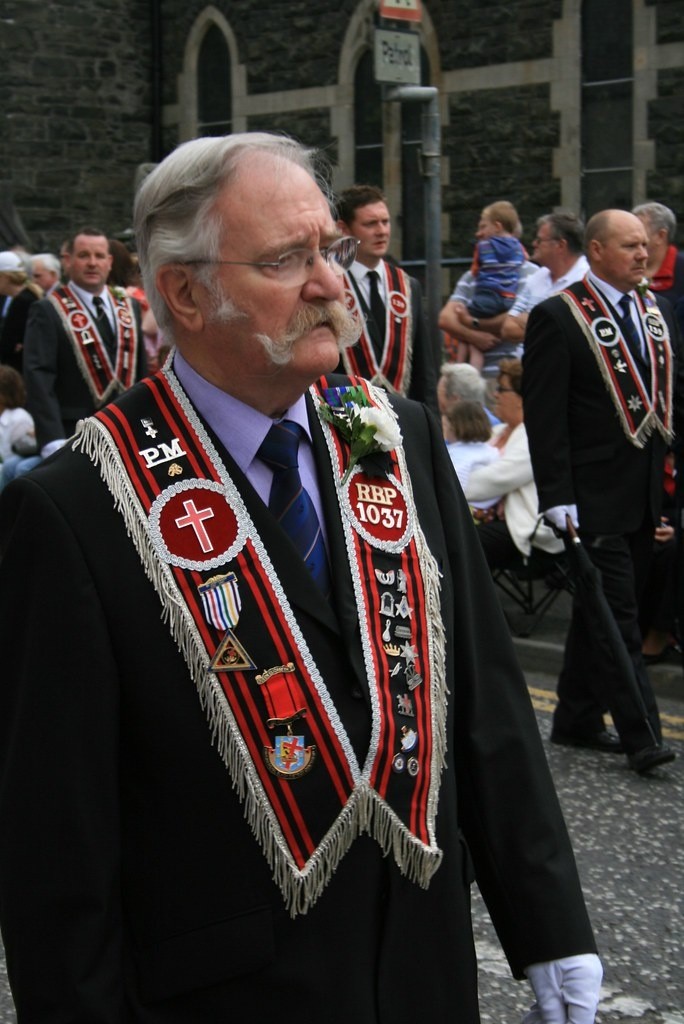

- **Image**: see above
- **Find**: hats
[1,250,28,274]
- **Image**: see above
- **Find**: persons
[0,184,684,774]
[0,133,604,1024]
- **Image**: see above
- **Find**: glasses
[535,235,551,246]
[174,236,361,278]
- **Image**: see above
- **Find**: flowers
[316,387,403,486]
[110,286,128,301]
[636,277,651,313]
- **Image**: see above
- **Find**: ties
[619,295,642,358]
[92,298,118,370]
[255,420,333,600]
[367,272,386,350]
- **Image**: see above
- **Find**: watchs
[473,317,480,329]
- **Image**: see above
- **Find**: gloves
[525,954,604,1024]
[544,505,579,530]
